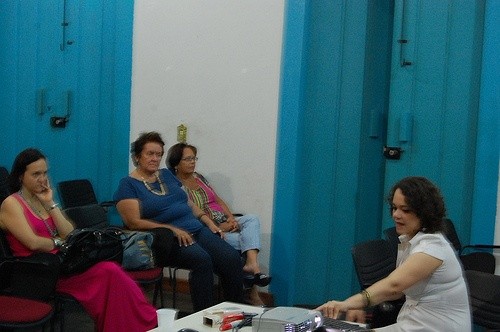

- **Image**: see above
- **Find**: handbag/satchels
[119,231,155,271]
[56,226,126,276]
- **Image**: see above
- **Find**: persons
[311,178,472,332]
[0,148,159,332]
[113,132,247,313]
[165,143,272,308]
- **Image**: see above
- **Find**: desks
[146,300,368,332]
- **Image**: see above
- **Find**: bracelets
[47,203,59,212]
[360,288,373,307]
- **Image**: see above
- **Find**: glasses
[180,156,199,161]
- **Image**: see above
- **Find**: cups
[156,309,177,332]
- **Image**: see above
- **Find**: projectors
[251,306,323,332]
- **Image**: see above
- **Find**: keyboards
[318,315,374,332]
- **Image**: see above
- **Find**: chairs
[0,179,247,332]
[351,218,500,332]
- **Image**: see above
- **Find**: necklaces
[138,172,166,196]
[19,190,58,237]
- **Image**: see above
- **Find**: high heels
[244,272,272,289]
